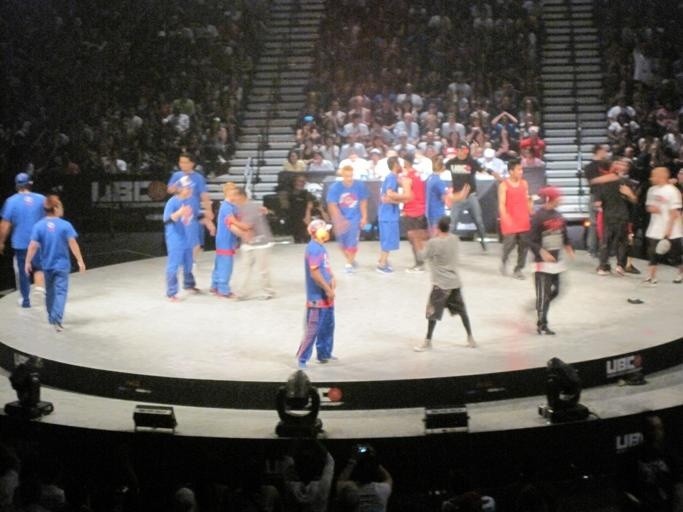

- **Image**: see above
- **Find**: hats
[484,148,494,157]
[455,140,466,149]
[174,175,196,189]
[339,159,353,169]
[307,219,332,234]
[15,173,33,186]
[655,235,671,255]
[538,188,558,201]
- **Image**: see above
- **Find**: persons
[641,166,683,286]
[208,182,269,300]
[583,141,644,277]
[442,141,503,252]
[498,157,533,281]
[278,175,322,243]
[604,26,683,167]
[161,175,217,302]
[293,220,338,369]
[166,152,214,271]
[282,0,377,171]
[24,195,86,333]
[375,157,410,273]
[517,187,577,336]
[476,149,504,180]
[326,159,369,275]
[379,152,426,272]
[0,1,267,180]
[1,408,683,511]
[0,173,47,308]
[520,147,546,169]
[425,156,470,237]
[226,186,278,303]
[377,0,543,156]
[408,216,477,350]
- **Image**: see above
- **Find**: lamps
[424,404,469,432]
[133,405,178,432]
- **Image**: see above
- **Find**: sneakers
[209,288,217,294]
[296,358,305,369]
[167,295,181,302]
[616,266,625,277]
[186,287,200,294]
[53,319,63,332]
[406,265,426,273]
[376,265,392,274]
[315,356,338,363]
[537,324,555,335]
[640,277,657,286]
[386,263,392,270]
[464,337,475,348]
[514,267,524,279]
[597,266,610,274]
[673,271,683,283]
[345,264,352,273]
[216,291,237,299]
[624,266,640,277]
[413,337,432,352]
[36,286,46,297]
